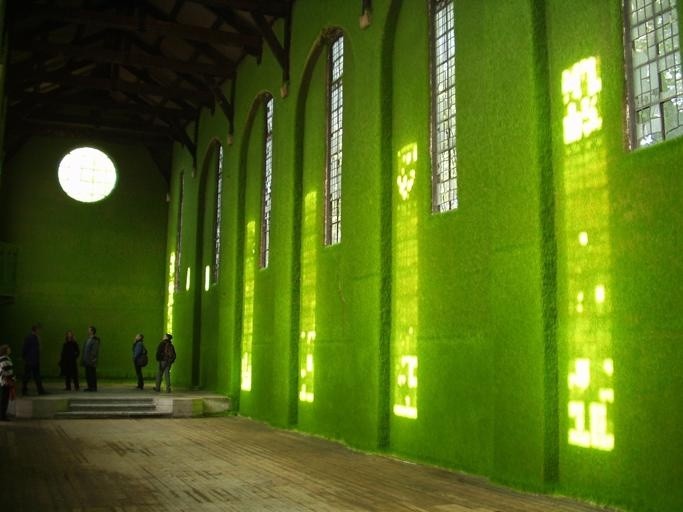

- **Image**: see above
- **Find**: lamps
[54,133,118,205]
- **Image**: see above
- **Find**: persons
[0,343,16,420]
[130,332,148,390]
[57,329,80,391]
[80,324,100,392]
[18,323,50,397]
[150,333,176,393]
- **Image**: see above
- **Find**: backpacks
[165,342,176,362]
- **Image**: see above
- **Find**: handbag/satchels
[136,354,146,366]
